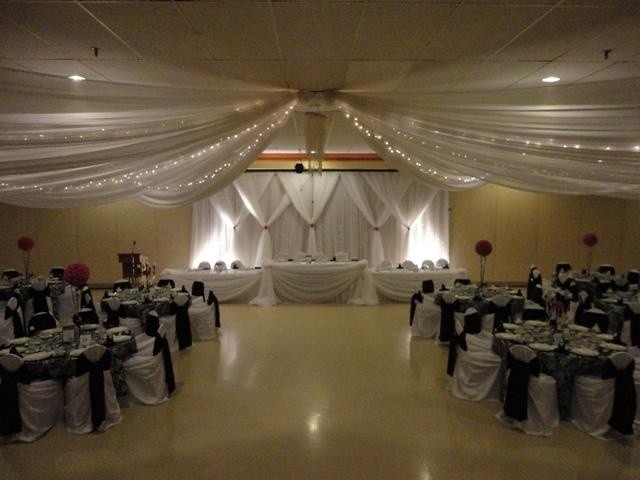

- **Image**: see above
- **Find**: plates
[102,286,189,305]
[449,285,517,299]
[0,323,132,361]
[495,319,626,357]
[0,280,63,290]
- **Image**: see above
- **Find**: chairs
[0,268,220,443]
